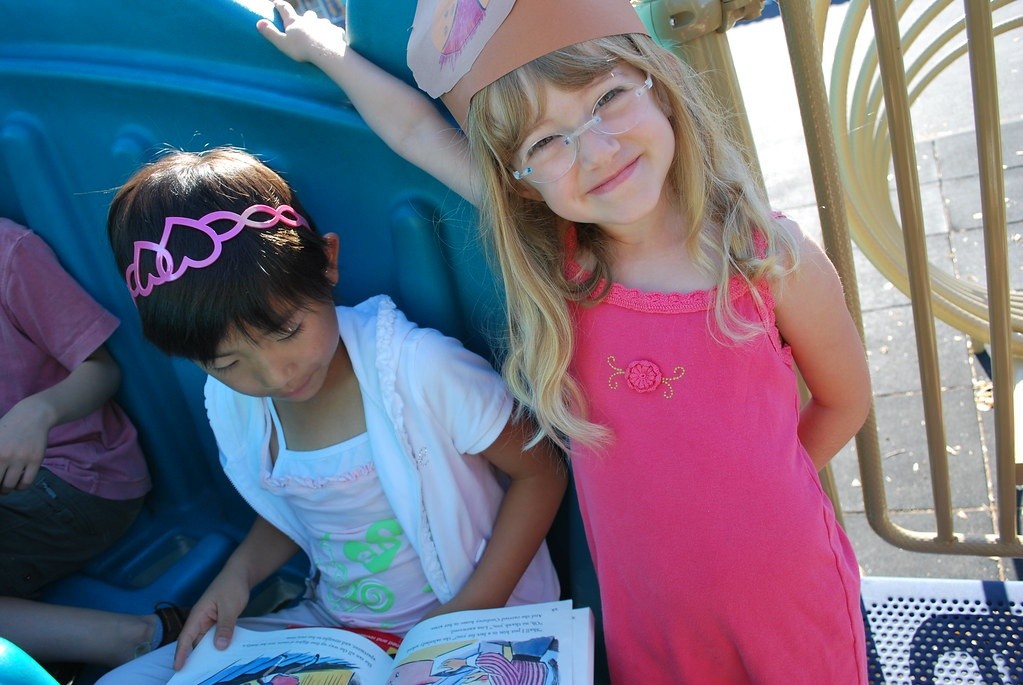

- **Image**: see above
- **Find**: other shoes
[153,601,192,650]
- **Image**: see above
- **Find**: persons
[256,0,872,685]
[0,220,184,665]
[108,149,566,669]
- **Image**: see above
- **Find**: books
[167,600,595,685]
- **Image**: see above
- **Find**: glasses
[506,69,653,184]
